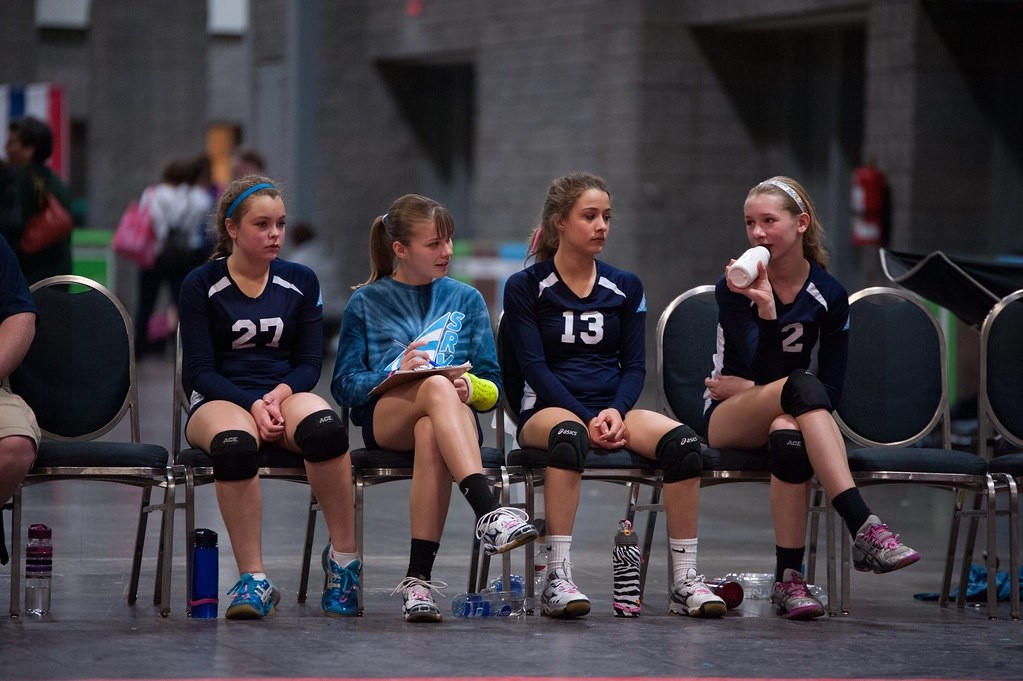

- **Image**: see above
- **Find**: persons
[328,193,540,622]
[116,120,265,352]
[702,175,921,617]
[496,172,728,616]
[0,118,73,297]
[0,236,41,567]
[181,175,362,619]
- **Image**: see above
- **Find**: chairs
[479,309,673,601]
[808,287,997,621]
[956,289,1023,620]
[4,275,175,618]
[641,284,837,616]
[340,404,511,616]
[153,320,317,614]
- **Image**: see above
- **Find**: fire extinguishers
[850,161,883,246]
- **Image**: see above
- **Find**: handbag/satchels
[15,170,73,259]
[110,183,158,268]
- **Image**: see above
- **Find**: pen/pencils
[390,338,439,367]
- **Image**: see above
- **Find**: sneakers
[541,558,591,619]
[321,540,363,618]
[851,522,921,575]
[225,572,281,621]
[389,577,449,623]
[668,566,727,619]
[475,507,539,556]
[767,568,825,620]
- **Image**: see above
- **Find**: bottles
[489,573,526,587]
[612,519,643,617]
[807,583,828,607]
[25,523,53,616]
[703,578,745,609]
[452,590,535,617]
[534,545,547,606]
[713,574,776,599]
[189,528,218,620]
[481,581,526,592]
[727,245,772,288]
